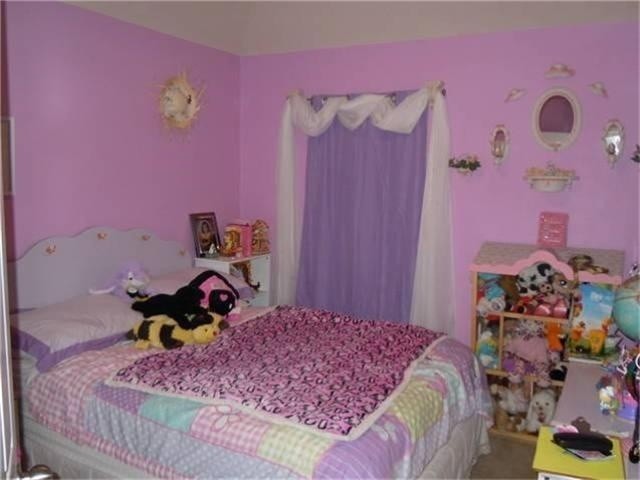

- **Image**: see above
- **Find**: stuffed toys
[131,286,208,330]
[476,272,576,434]
[89,258,156,303]
[126,306,239,348]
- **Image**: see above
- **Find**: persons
[224,231,237,249]
[199,220,215,251]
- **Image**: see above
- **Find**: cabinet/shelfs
[474,241,629,439]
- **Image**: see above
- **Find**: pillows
[11,261,259,373]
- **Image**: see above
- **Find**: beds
[10,226,488,480]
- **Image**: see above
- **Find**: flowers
[448,150,481,171]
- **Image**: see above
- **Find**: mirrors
[603,122,624,167]
[489,127,510,162]
[533,87,581,150]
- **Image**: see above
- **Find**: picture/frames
[188,211,223,257]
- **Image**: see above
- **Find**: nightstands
[193,255,270,306]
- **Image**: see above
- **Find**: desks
[534,356,640,480]
